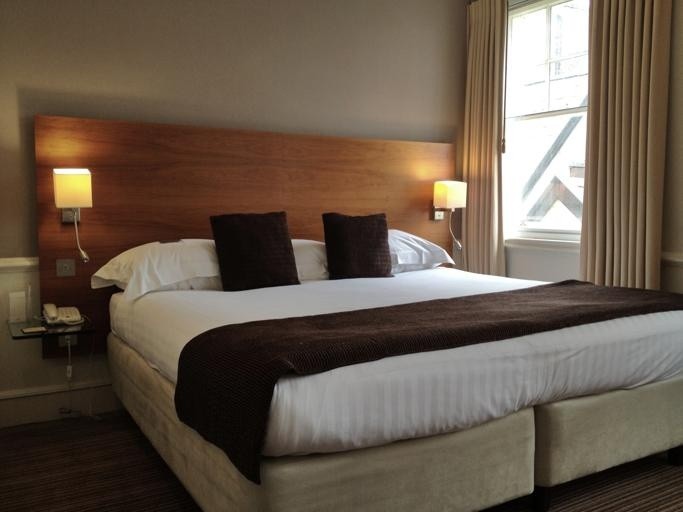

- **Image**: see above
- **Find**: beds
[31,112,683,512]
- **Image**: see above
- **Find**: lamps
[53,168,93,261]
[432,180,467,250]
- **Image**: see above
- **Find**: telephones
[43,304,84,324]
